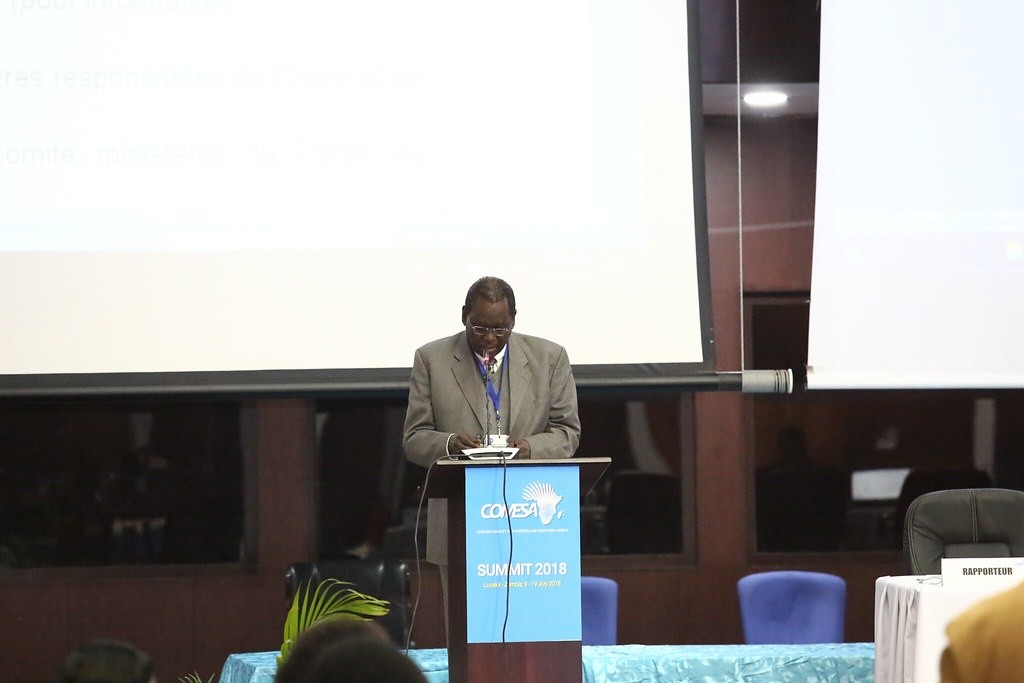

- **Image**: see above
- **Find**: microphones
[459,350,520,460]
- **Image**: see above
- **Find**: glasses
[466,310,512,336]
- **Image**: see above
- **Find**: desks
[217,645,874,683]
[875,574,1024,683]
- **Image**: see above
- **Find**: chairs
[581,576,620,645]
[901,487,1024,574]
[738,570,848,644]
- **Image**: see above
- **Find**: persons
[402,276,581,652]
[274,618,433,683]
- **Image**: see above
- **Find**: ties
[484,359,496,380]
